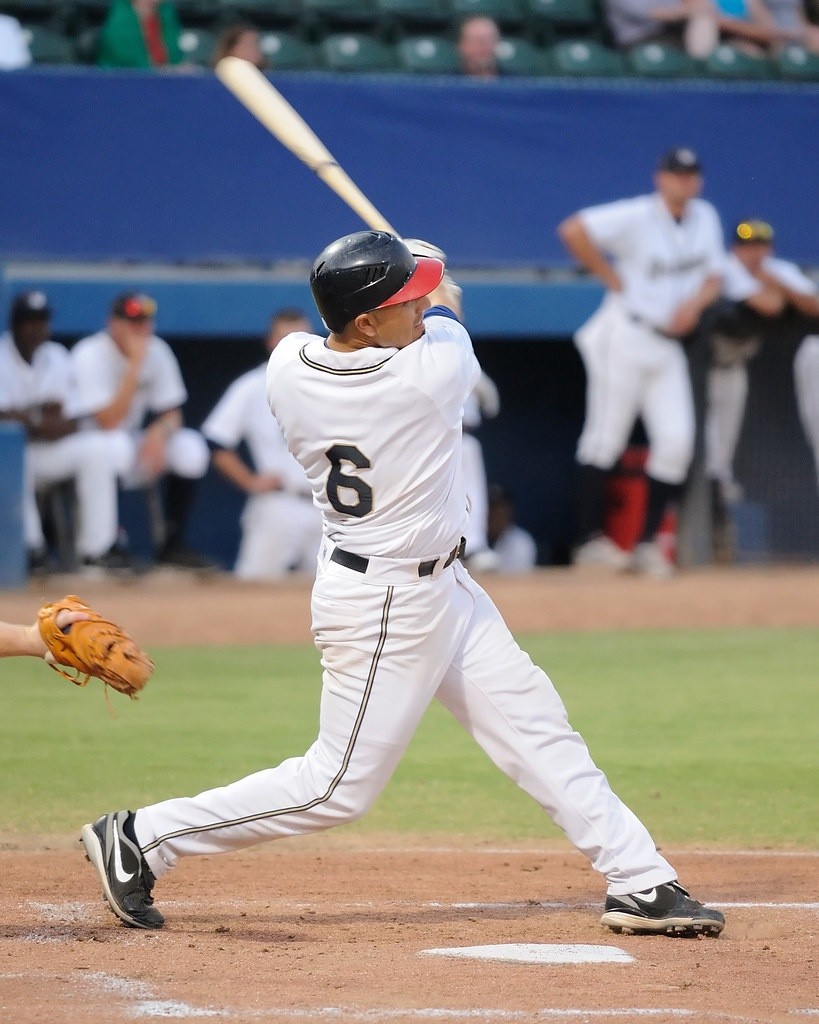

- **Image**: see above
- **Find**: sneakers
[78,808,166,930]
[601,878,725,937]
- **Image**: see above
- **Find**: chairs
[1,0,819,84]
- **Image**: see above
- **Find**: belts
[330,537,467,575]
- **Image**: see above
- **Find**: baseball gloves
[37,594,157,695]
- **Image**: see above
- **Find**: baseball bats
[214,55,403,240]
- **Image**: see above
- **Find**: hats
[662,148,699,179]
[113,295,156,322]
[14,292,53,319]
[736,222,781,250]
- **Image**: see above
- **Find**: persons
[0,594,154,701]
[200,309,322,581]
[209,24,272,71]
[0,14,34,71]
[441,15,541,76]
[0,290,210,586]
[558,148,818,579]
[78,230,725,936]
[459,369,537,573]
[605,0,819,60]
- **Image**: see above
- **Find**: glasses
[739,225,775,242]
[121,300,155,320]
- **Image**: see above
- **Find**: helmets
[309,229,448,331]
